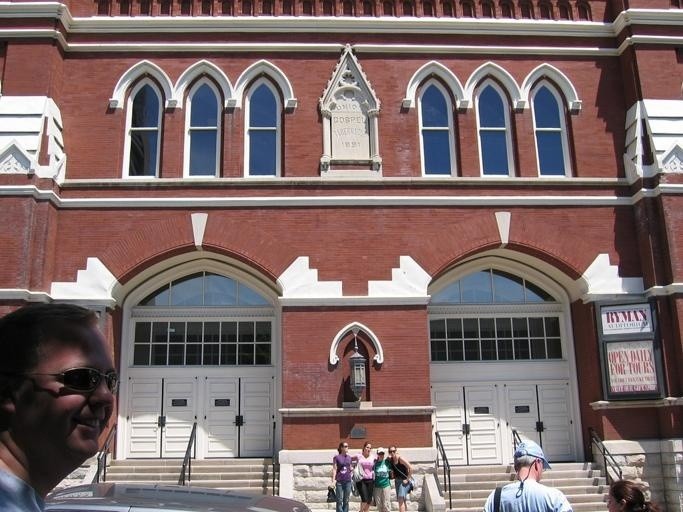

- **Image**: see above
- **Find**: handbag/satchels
[407,477,415,493]
[327,485,337,502]
[351,464,363,496]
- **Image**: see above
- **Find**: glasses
[342,446,349,448]
[25,367,119,392]
[389,450,396,453]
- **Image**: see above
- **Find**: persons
[606,480,655,512]
[331,441,412,512]
[485,441,573,512]
[0,302,118,512]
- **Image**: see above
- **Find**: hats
[513,440,551,469]
[377,447,385,454]
